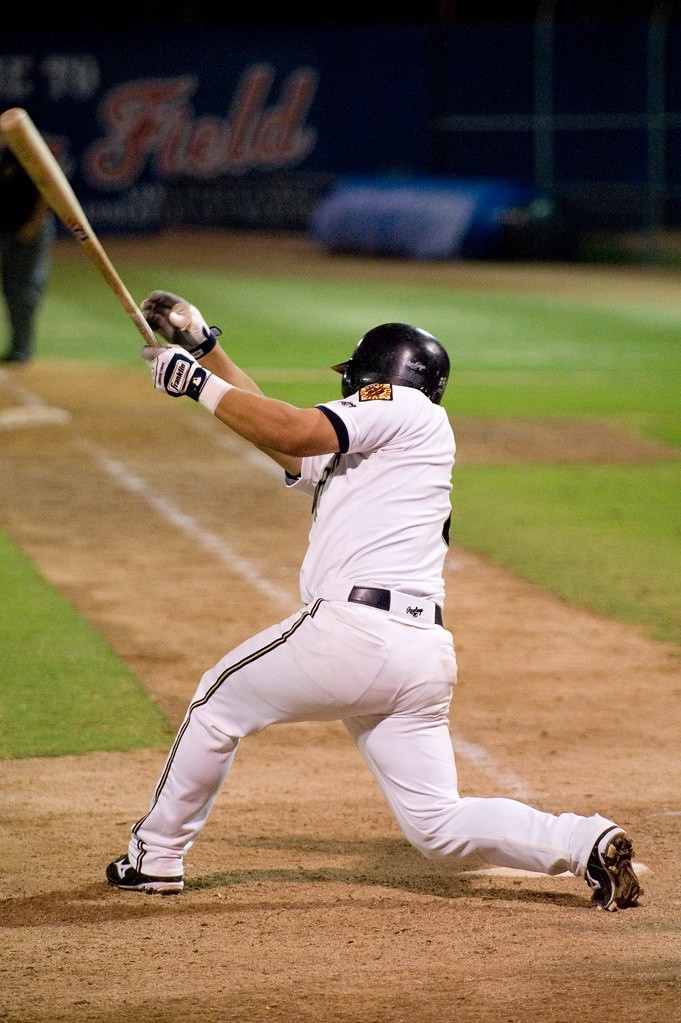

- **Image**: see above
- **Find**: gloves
[140,290,222,360]
[140,342,233,417]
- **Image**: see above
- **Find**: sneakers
[106,854,184,894]
[586,826,640,911]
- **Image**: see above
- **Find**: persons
[106,290,640,911]
[0,146,54,364]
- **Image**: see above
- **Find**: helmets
[330,323,451,405]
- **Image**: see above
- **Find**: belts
[349,586,444,626]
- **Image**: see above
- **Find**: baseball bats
[0,104,160,347]
